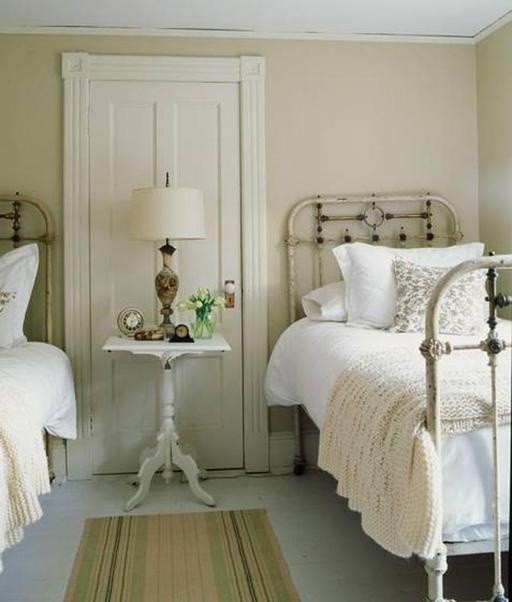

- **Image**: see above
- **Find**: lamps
[126,172,205,337]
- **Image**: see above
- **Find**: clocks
[118,306,144,336]
[170,324,194,343]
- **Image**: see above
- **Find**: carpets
[58,508,303,602]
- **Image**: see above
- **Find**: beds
[260,191,512,601]
[0,192,80,601]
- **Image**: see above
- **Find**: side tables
[100,334,233,512]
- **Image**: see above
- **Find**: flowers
[178,284,227,339]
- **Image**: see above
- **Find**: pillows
[0,243,40,350]
[299,280,346,322]
[331,241,483,330]
[389,255,490,340]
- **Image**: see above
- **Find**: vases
[189,314,217,339]
